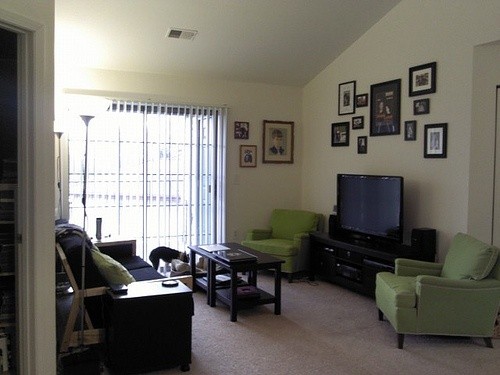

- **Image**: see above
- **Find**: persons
[376,101,395,133]
[269,129,287,156]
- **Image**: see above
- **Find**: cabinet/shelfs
[308,232,437,297]
[0,181,19,374]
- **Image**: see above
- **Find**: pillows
[440,232,500,280]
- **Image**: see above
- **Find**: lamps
[55,131,68,224]
[61,113,102,375]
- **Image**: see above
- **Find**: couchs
[57,224,196,375]
[240,208,324,282]
[378,250,500,349]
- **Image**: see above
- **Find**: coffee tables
[191,242,286,323]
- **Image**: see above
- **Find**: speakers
[328,214,336,239]
[412,228,436,257]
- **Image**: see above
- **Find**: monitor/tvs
[337,173,403,246]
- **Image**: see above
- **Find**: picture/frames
[330,76,368,155]
[369,77,401,136]
[405,119,416,141]
[408,62,437,97]
[414,97,431,115]
[233,120,249,139]
[239,144,257,167]
[423,122,449,159]
[262,120,296,164]
